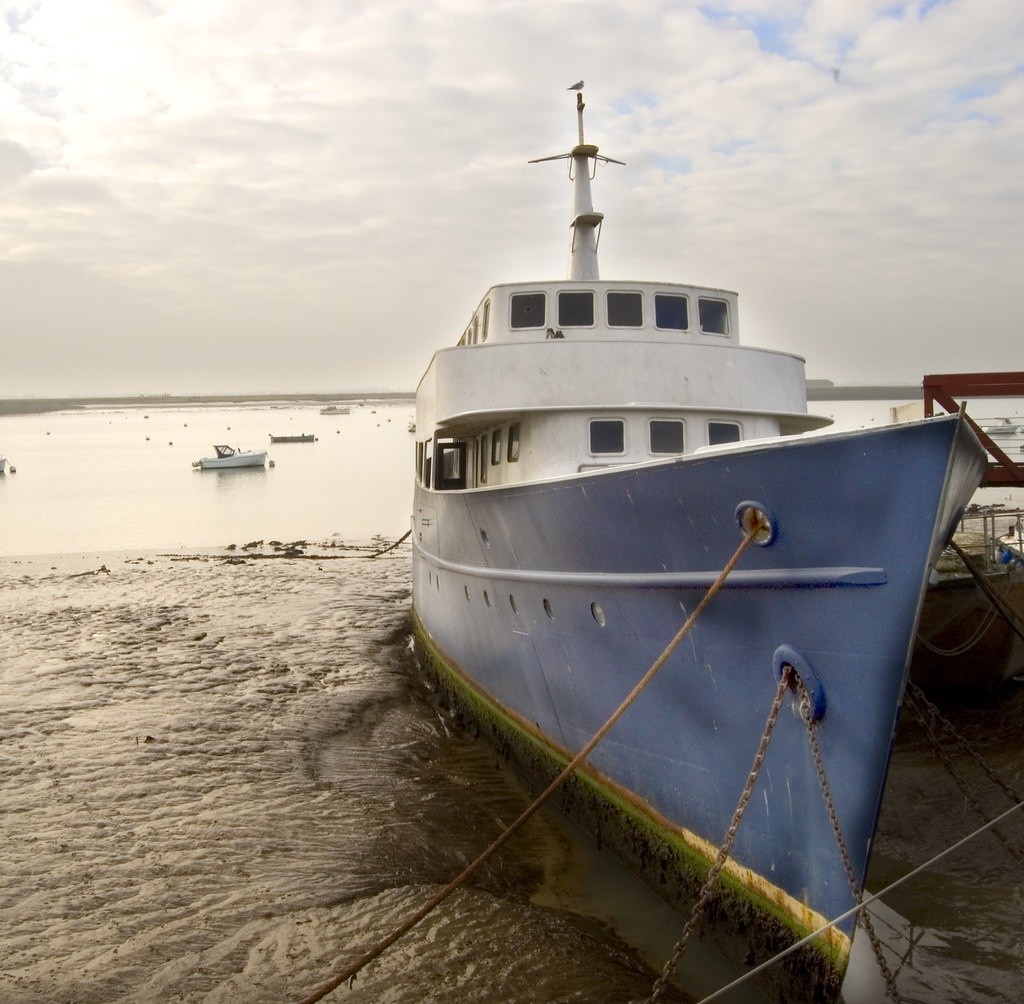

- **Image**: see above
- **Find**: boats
[321,404,352,417]
[981,424,1019,434]
[411,82,998,1004]
[0,456,8,473]
[192,443,269,470]
[268,434,316,442]
